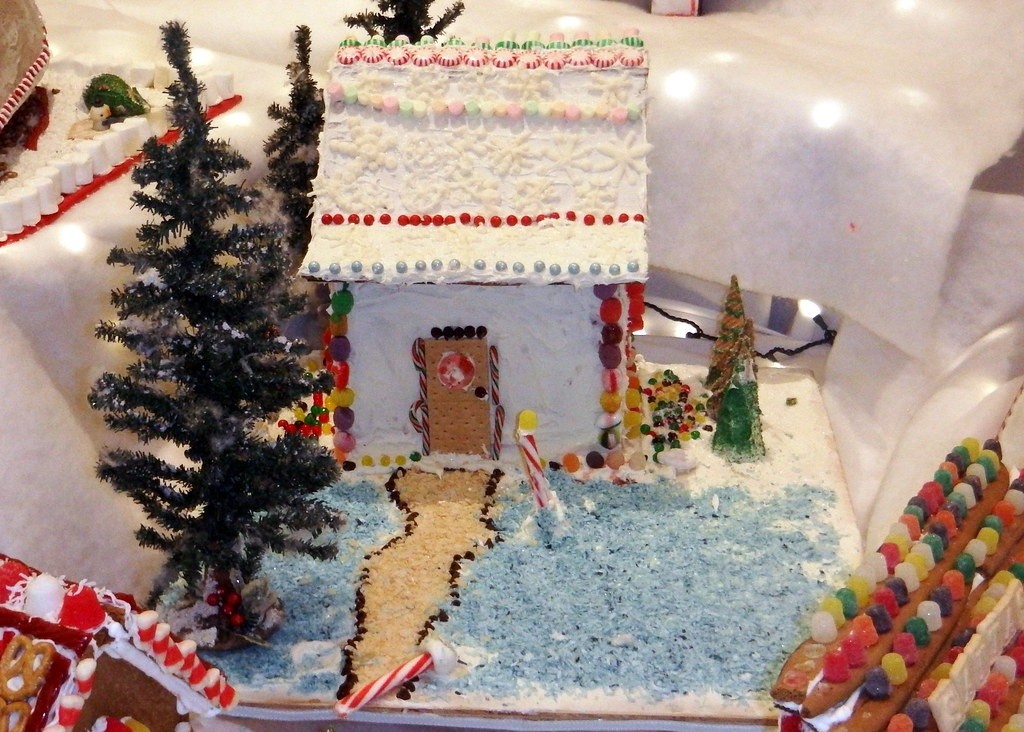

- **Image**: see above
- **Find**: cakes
[297,31,650,490]
[0,553,239,732]
[0,0,55,176]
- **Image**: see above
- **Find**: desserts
[776,431,1024,729]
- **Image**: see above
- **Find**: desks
[108,0,1024,360]
